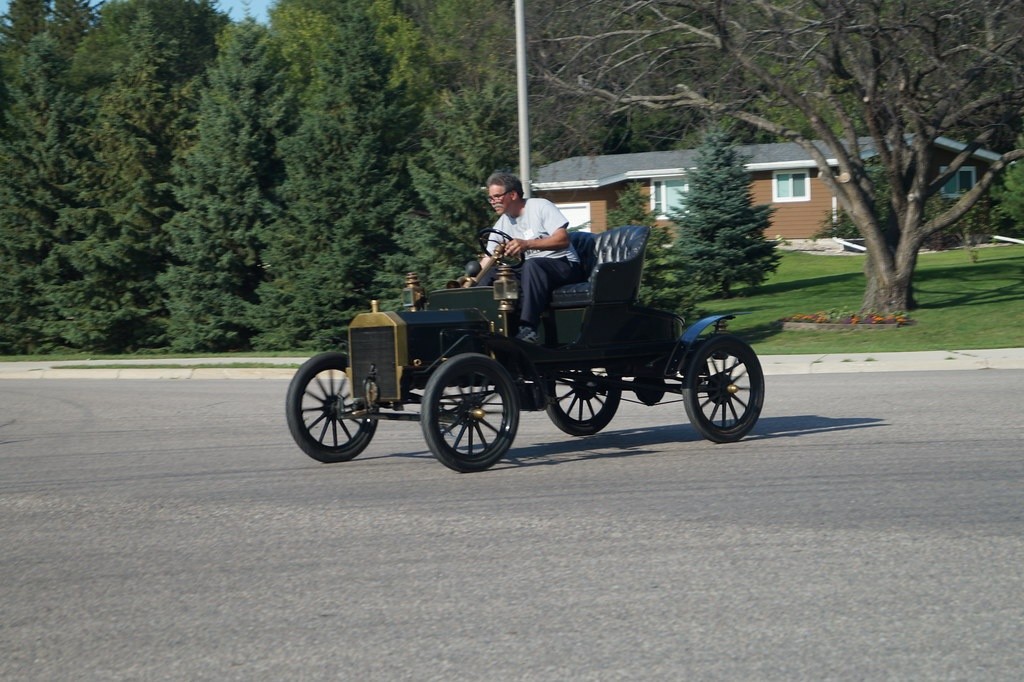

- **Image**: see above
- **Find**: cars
[283,217,766,475]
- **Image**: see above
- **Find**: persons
[458,171,582,347]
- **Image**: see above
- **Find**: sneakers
[515,325,542,346]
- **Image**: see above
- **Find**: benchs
[549,225,649,307]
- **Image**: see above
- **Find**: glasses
[486,190,511,204]
